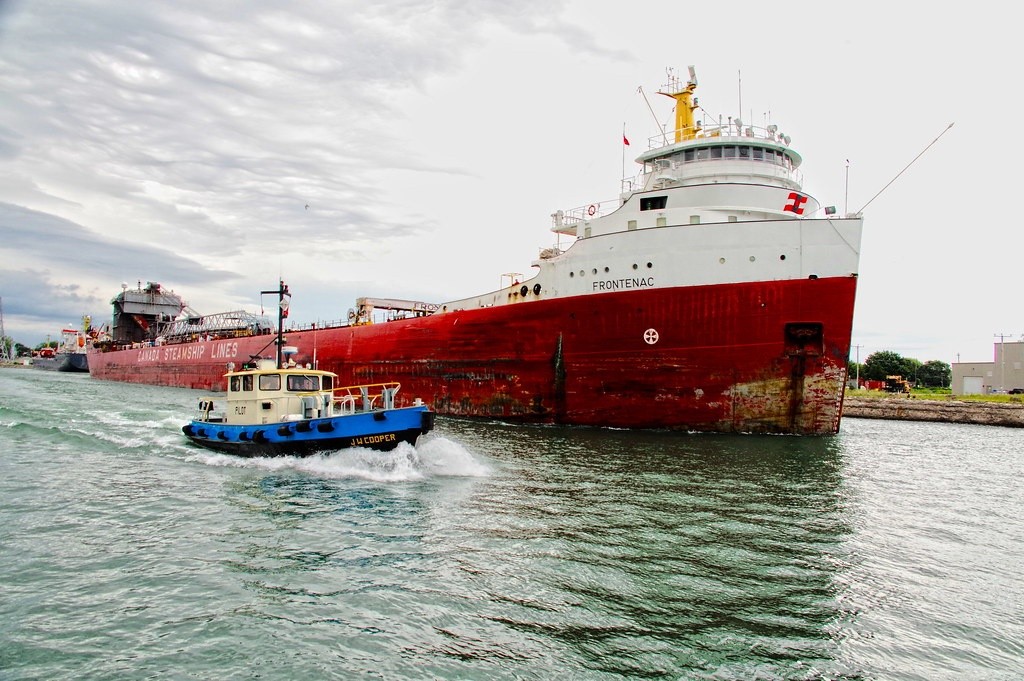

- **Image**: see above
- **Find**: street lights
[851,344,864,389]
[994,333,1012,393]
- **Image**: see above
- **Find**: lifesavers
[587,204,596,217]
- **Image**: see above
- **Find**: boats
[33,323,90,373]
[182,277,437,458]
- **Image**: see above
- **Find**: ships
[85,66,954,436]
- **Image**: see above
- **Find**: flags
[624,137,630,145]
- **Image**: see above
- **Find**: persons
[513,279,519,285]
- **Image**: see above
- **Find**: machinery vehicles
[882,375,911,394]
